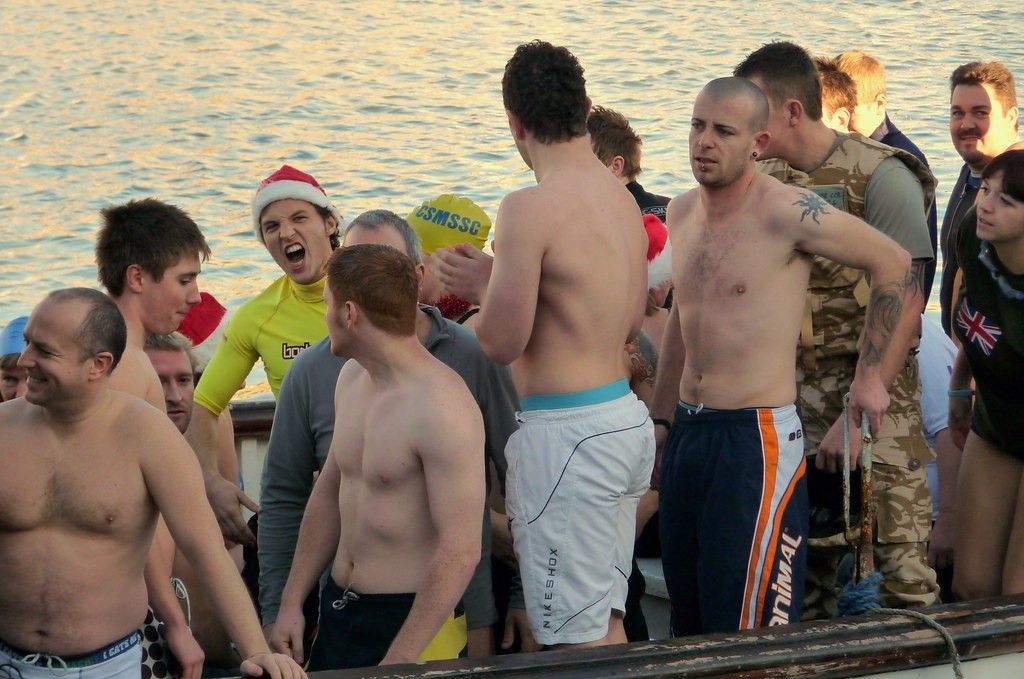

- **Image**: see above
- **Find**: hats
[252,165,342,245]
[0,317,28,359]
[642,211,672,291]
[405,194,491,262]
[175,292,230,375]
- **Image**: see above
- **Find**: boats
[211,392,1024,679]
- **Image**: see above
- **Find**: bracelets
[948,389,972,398]
[653,418,671,430]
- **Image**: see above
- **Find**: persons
[428,39,657,653]
[0,40,1024,679]
